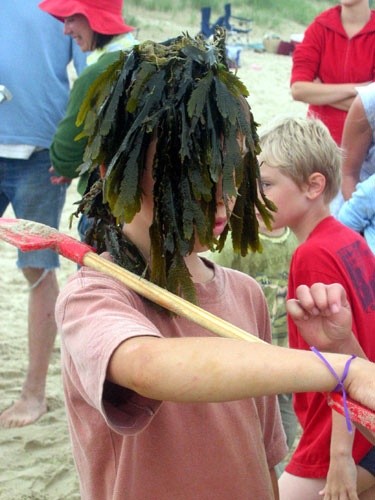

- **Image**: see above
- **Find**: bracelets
[309,346,357,431]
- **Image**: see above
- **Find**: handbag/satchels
[255,36,299,55]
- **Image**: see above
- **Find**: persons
[254,0,374,500]
[53,34,374,500]
[0,0,141,429]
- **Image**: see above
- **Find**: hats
[38,0,135,35]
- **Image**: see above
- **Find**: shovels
[0,217,375,448]
